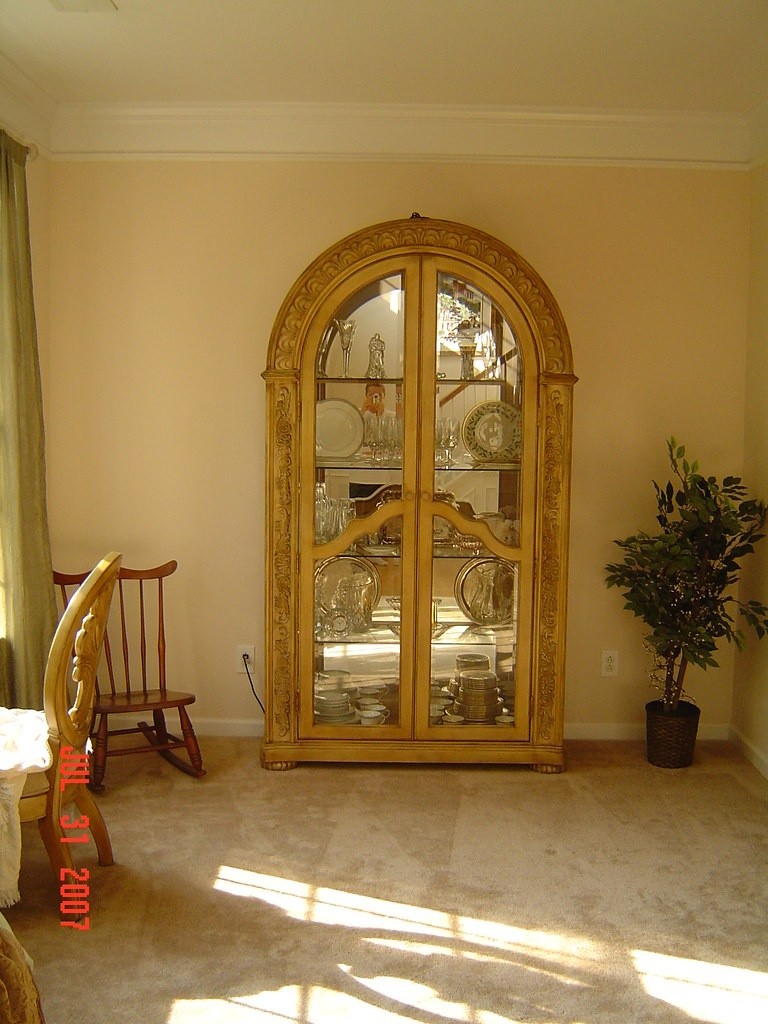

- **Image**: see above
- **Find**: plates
[314,557,382,613]
[441,653,509,725]
[315,398,364,458]
[462,400,522,463]
[454,557,517,626]
[314,670,361,723]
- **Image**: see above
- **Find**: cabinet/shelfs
[262,217,576,771]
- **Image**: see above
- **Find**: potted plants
[604,438,768,769]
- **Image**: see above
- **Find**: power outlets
[237,645,254,673]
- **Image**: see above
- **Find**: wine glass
[334,319,358,379]
[364,416,402,463]
[435,417,460,468]
[480,336,491,381]
[490,336,498,380]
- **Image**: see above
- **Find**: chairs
[51,561,205,795]
[0,550,123,922]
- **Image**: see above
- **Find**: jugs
[337,578,373,632]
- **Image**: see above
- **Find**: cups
[315,482,356,554]
[459,344,476,379]
[357,684,391,725]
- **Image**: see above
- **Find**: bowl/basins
[389,623,450,638]
